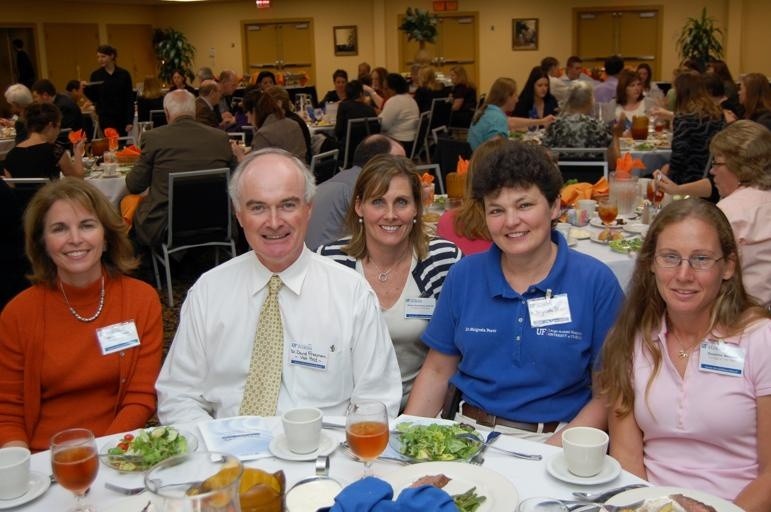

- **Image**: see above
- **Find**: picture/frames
[331,25,358,57]
[510,16,539,51]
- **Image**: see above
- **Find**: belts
[461,404,558,434]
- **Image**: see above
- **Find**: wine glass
[344,397,388,477]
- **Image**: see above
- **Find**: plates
[104,172,121,178]
[390,419,486,460]
[312,123,335,128]
[0,469,50,511]
[619,129,673,157]
[555,176,666,246]
[268,429,339,461]
[100,427,199,474]
[384,461,519,512]
[601,486,751,512]
[118,168,130,174]
[421,211,442,226]
[545,450,623,485]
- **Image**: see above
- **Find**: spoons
[572,483,644,501]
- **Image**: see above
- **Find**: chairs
[1,74,698,309]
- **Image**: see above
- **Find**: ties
[240,275,284,416]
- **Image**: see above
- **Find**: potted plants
[397,7,439,85]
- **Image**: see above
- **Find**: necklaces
[368,251,406,283]
[60,275,105,322]
[666,319,703,358]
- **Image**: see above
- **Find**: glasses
[652,253,724,272]
[710,160,723,170]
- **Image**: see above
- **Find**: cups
[103,164,117,175]
[631,115,650,140]
[89,138,107,167]
[283,456,345,511]
[568,501,609,512]
[445,173,466,202]
[49,429,100,511]
[146,450,244,511]
[282,406,324,453]
[190,469,286,511]
[445,197,464,212]
[562,426,611,475]
[1,446,32,500]
[421,183,435,215]
[513,497,573,512]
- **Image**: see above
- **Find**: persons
[2,46,134,179]
[599,197,771,512]
[651,61,771,199]
[319,62,478,168]
[468,77,555,152]
[154,146,404,424]
[707,118,771,317]
[512,66,560,129]
[1,175,166,455]
[398,138,628,449]
[542,55,666,169]
[314,153,466,420]
[169,67,312,166]
[126,88,237,250]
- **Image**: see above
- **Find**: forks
[342,442,408,467]
[455,431,543,461]
[104,479,195,497]
[469,430,500,467]
